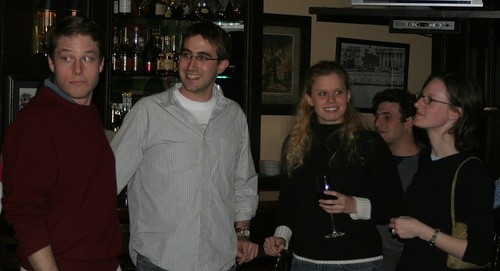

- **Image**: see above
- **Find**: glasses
[415,91,457,107]
[179,51,224,65]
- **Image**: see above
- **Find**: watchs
[427,228,439,247]
[236,228,252,239]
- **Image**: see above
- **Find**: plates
[260,160,281,175]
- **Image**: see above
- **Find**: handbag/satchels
[448,156,500,269]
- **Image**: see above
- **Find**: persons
[1,14,124,271]
[111,21,259,271]
[263,60,500,271]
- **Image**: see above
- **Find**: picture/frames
[261,13,314,114]
[336,37,410,113]
[5,75,51,127]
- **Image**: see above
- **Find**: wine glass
[315,174,345,238]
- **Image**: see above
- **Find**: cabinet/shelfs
[4,0,265,160]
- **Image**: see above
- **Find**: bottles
[111,0,247,20]
[112,26,182,77]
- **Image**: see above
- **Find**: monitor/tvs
[351,0,484,9]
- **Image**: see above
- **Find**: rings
[392,229,396,234]
